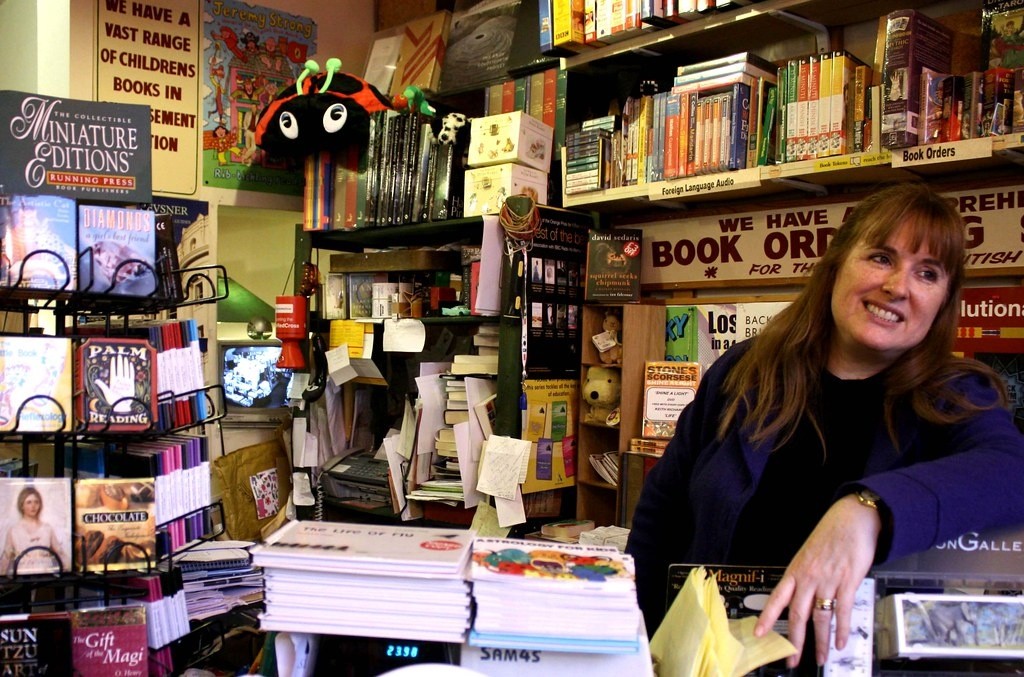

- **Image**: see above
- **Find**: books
[0,315,208,432]
[405,322,500,504]
[487,68,565,160]
[565,1,1024,197]
[0,539,265,677]
[590,451,620,486]
[624,187,1024,666]
[250,520,642,655]
[0,192,188,303]
[0,431,213,576]
[303,112,470,232]
[538,0,749,56]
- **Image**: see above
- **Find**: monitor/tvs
[217,338,293,429]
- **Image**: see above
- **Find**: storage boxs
[468,110,554,173]
[877,592,1024,661]
[463,162,547,217]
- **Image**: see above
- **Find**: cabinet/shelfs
[293,223,525,518]
[557,0,1024,216]
[576,303,666,528]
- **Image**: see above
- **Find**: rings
[814,599,837,610]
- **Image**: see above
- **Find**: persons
[1,487,71,575]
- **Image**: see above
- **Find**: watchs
[855,490,891,561]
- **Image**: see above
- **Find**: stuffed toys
[255,58,436,155]
[581,367,622,425]
[595,315,624,363]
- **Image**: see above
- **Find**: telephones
[318,445,390,487]
